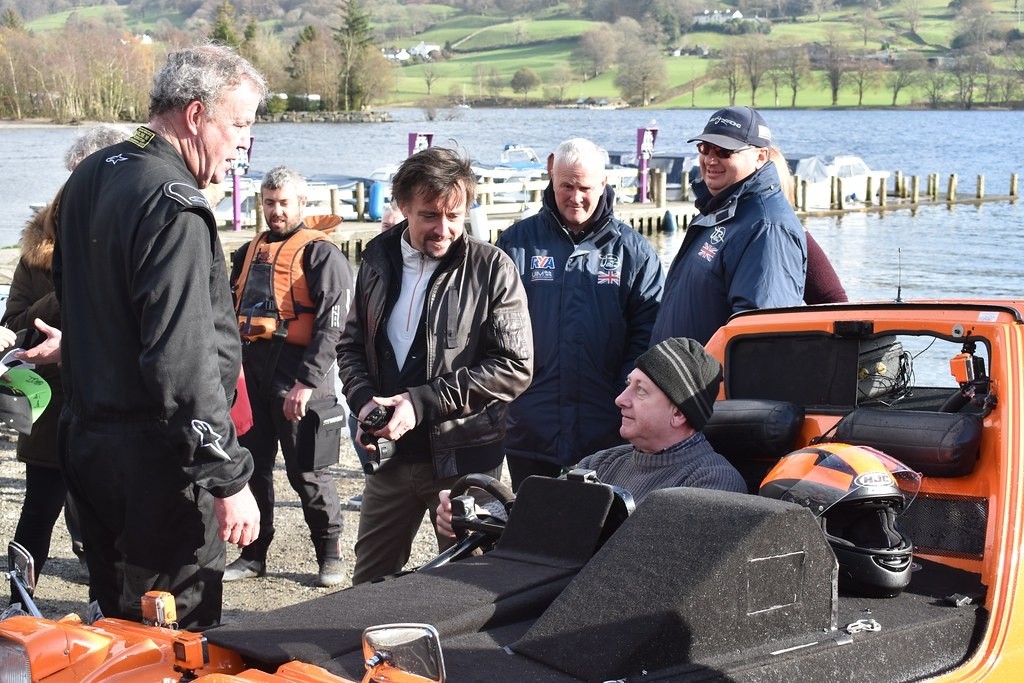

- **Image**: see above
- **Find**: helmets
[759,443,921,596]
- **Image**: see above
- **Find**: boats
[206,119,895,232]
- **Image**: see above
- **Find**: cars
[0,296,1024,683]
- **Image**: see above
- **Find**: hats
[686,106,771,151]
[634,337,723,430]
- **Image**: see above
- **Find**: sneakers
[320,558,346,586]
[221,557,264,580]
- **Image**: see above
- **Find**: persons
[0,124,133,624]
[336,147,534,586]
[769,146,849,305]
[495,138,666,493]
[649,106,808,351]
[222,166,354,587]
[437,337,749,538]
[50,46,270,629]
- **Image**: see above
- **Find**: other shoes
[0,603,30,622]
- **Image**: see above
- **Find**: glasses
[697,143,756,159]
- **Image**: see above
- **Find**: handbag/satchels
[297,400,346,470]
